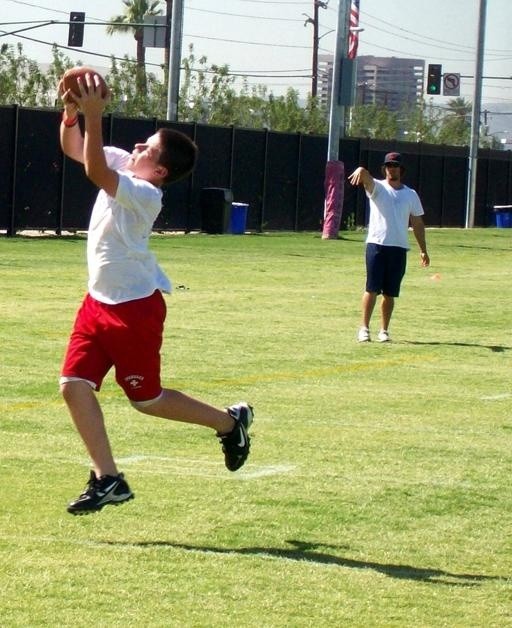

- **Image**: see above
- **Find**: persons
[346,151,430,343]
[53,66,257,517]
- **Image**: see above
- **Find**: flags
[347,0,360,59]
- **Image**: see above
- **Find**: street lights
[311,26,365,107]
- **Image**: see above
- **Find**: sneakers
[377,330,392,341]
[66,468,134,516]
[358,327,369,342]
[217,401,254,471]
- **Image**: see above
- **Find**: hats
[382,152,403,165]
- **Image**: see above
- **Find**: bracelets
[61,111,80,128]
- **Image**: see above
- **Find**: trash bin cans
[199,188,249,235]
[493,205,512,228]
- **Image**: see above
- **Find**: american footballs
[62,66,109,105]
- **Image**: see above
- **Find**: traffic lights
[426,64,442,96]
